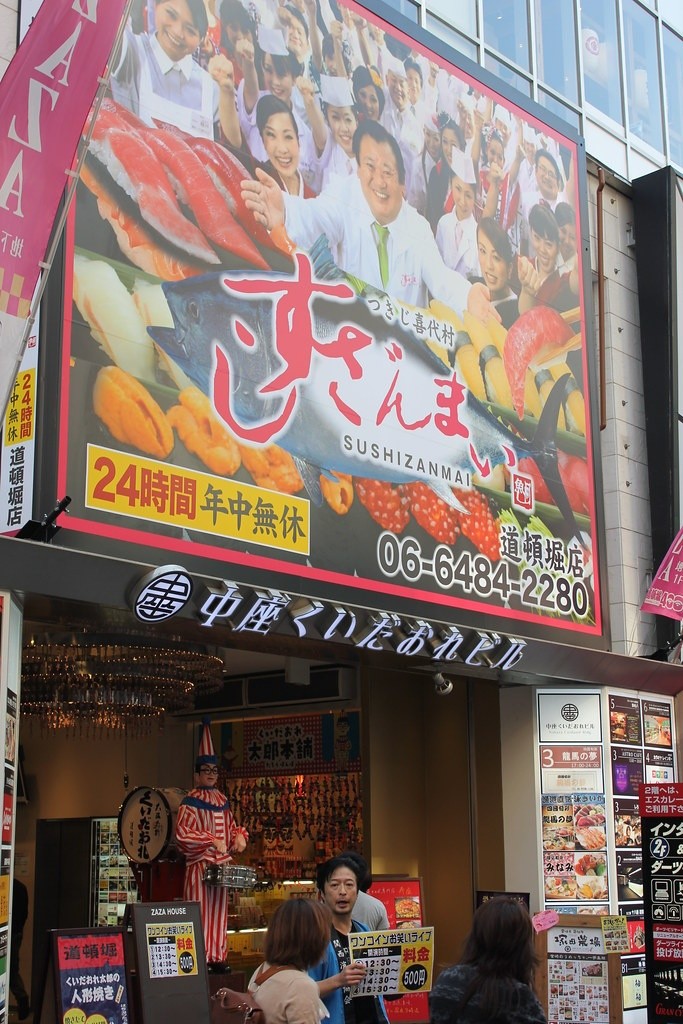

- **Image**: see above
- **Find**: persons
[175,755,249,973]
[9,859,30,1020]
[427,896,547,1024]
[247,899,333,1024]
[105,0,583,398]
[614,815,642,847]
[307,857,392,1024]
[338,850,390,932]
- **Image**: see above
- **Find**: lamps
[432,673,453,695]
[20,627,227,739]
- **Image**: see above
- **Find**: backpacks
[209,961,301,1024]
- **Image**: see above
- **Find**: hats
[451,145,477,184]
[257,25,290,57]
[388,55,407,78]
[319,73,354,107]
[477,95,557,151]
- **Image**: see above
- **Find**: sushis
[402,296,587,509]
[69,96,299,385]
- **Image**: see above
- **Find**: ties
[374,222,389,290]
[455,224,462,247]
[397,112,402,128]
